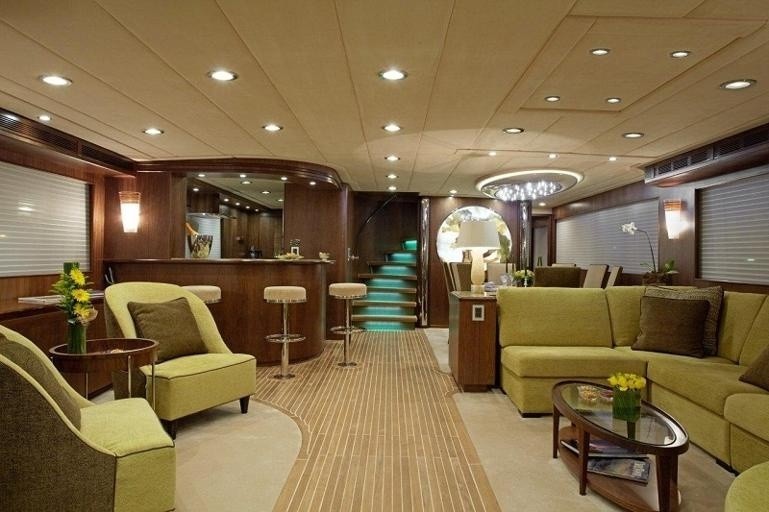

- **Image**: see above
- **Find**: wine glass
[499,273,516,286]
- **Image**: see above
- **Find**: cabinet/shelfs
[448,290,497,393]
[0,287,114,401]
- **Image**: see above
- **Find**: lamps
[453,219,501,294]
[469,168,582,204]
[660,197,685,240]
[117,188,143,236]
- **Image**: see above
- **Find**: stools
[181,284,220,304]
[326,282,369,370]
[261,282,309,381]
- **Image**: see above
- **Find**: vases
[612,387,643,421]
[640,272,672,285]
[67,320,87,354]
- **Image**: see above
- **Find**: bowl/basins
[577,385,602,401]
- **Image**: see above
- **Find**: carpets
[452,387,740,512]
[422,325,452,376]
[85,382,304,511]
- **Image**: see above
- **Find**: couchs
[721,457,769,512]
[102,281,259,442]
[494,280,769,477]
[0,322,179,512]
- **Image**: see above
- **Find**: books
[558,435,654,485]
[17,289,105,307]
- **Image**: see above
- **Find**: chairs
[441,253,623,336]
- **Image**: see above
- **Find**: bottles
[537,256,542,266]
[184,222,199,235]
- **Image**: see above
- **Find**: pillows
[1,339,84,434]
[629,295,711,359]
[641,285,724,358]
[125,297,210,363]
[736,343,768,391]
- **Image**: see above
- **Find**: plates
[274,255,305,259]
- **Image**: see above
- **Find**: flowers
[50,262,100,352]
[619,221,680,277]
[608,371,647,422]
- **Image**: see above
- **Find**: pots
[249,249,262,258]
